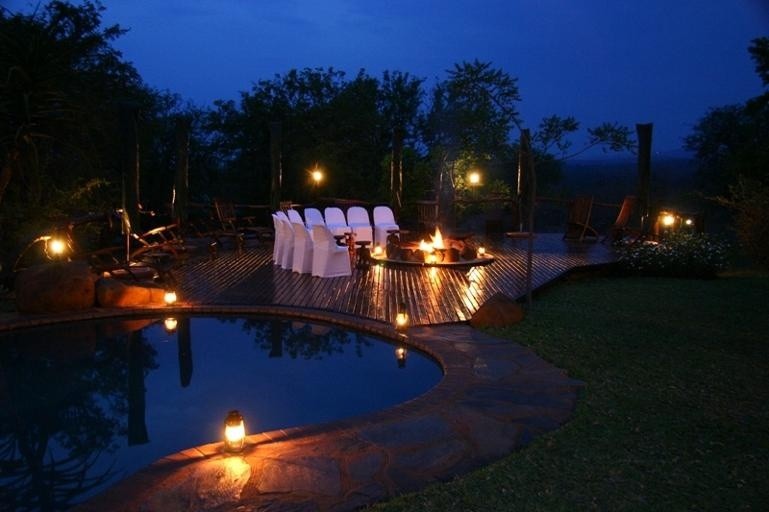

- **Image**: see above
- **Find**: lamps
[224,410,248,452]
[395,302,410,330]
[478,242,486,256]
[395,347,408,369]
[164,317,178,333]
[374,242,383,254]
[164,288,177,306]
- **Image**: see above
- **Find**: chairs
[292,224,313,274]
[601,208,632,245]
[311,224,352,277]
[272,214,283,260]
[276,211,289,223]
[281,220,294,269]
[347,206,373,248]
[115,208,186,262]
[373,206,400,247]
[304,208,324,227]
[561,195,599,243]
[287,209,304,226]
[209,199,265,256]
[325,207,351,243]
